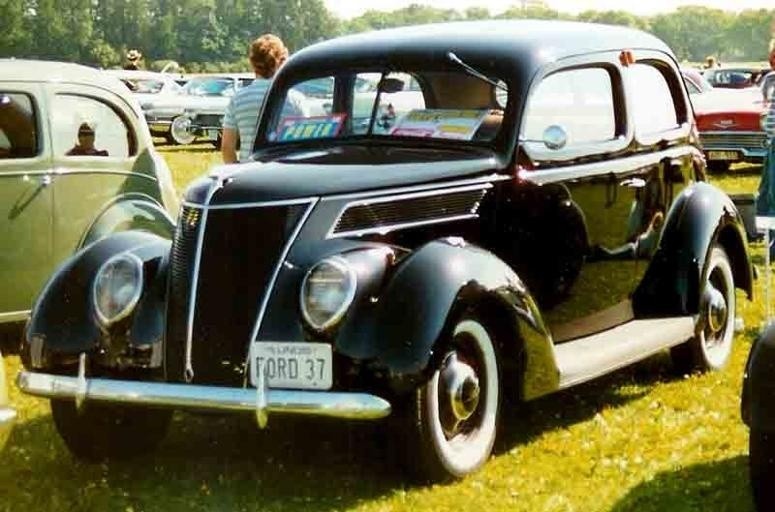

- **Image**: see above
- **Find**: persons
[704,57,714,68]
[125,49,141,89]
[222,34,308,162]
[760,41,774,136]
[67,123,109,156]
